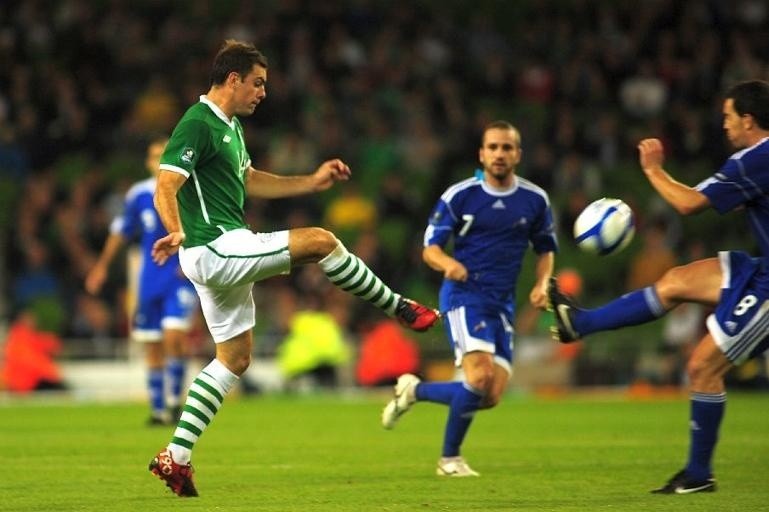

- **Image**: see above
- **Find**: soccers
[572,198,636,257]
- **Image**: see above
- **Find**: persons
[380,117,560,478]
[541,79,769,499]
[1,2,769,341]
[144,39,443,500]
[84,136,201,424]
[274,291,351,389]
[2,303,72,390]
[354,316,430,387]
[630,344,689,386]
[722,352,769,390]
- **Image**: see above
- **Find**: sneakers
[149,448,199,498]
[380,374,421,430]
[436,455,480,478]
[650,472,717,496]
[396,297,437,331]
[546,278,579,343]
